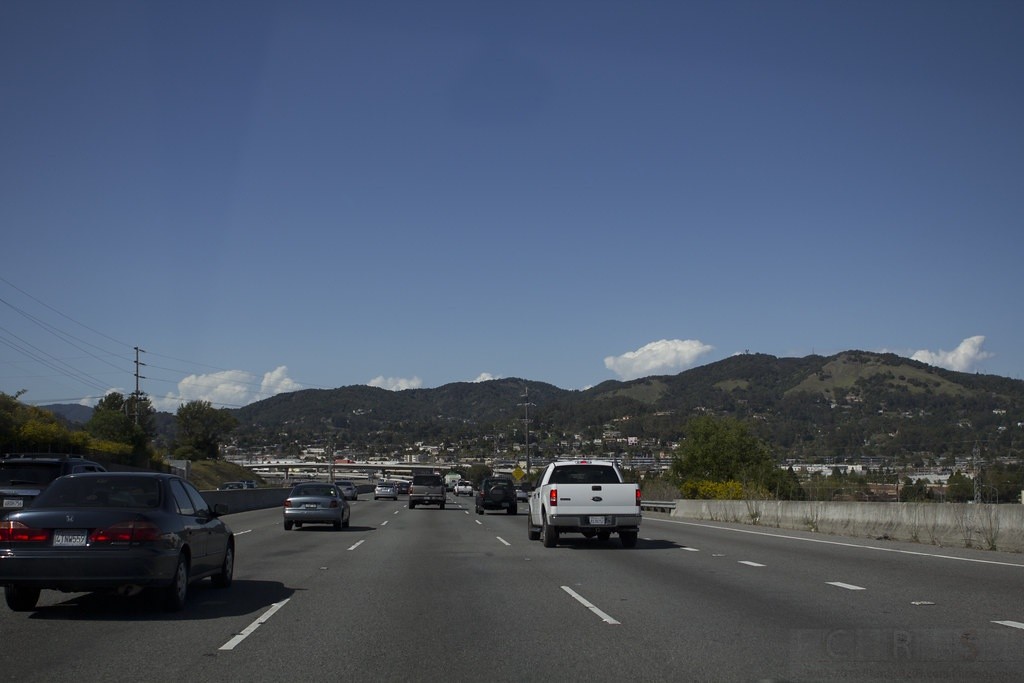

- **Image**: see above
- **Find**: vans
[445,473,461,491]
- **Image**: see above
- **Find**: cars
[513,485,527,502]
[283,483,351,530]
[217,481,257,490]
[334,480,409,502]
[2,473,237,608]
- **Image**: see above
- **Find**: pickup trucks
[452,480,473,496]
[527,458,641,547]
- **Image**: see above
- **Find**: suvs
[408,474,446,510]
[1,451,108,516]
[475,477,517,515]
